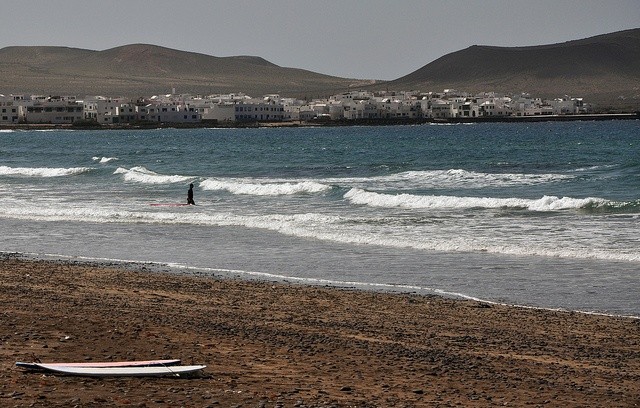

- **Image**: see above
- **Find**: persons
[187,184,195,205]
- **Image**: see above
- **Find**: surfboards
[151,204,191,207]
[36,362,207,379]
[16,356,183,368]
